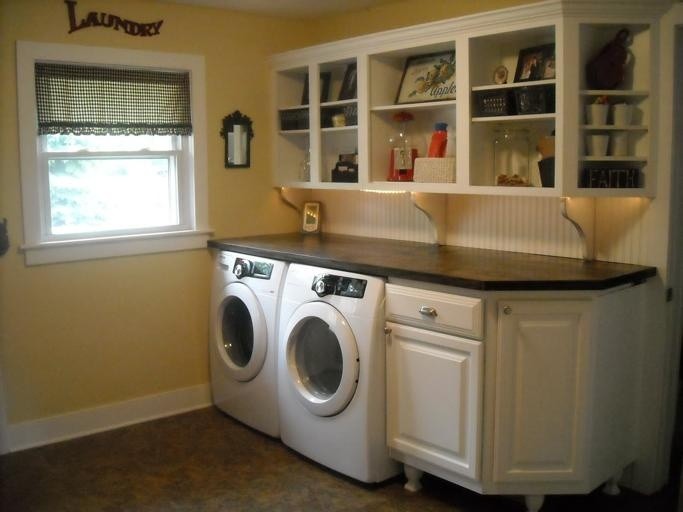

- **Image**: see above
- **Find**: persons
[529,56,539,80]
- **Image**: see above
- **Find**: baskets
[481,92,508,116]
[515,87,547,114]
[413,158,454,182]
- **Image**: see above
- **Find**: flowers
[393,112,415,166]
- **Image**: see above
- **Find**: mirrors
[220,109,256,168]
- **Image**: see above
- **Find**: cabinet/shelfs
[491,276,660,496]
[272,1,660,198]
[384,275,488,494]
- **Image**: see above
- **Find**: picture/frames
[512,43,555,83]
[301,63,356,105]
[393,50,455,105]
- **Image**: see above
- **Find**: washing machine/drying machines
[207,249,289,444]
[277,262,403,485]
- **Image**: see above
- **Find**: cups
[586,104,635,155]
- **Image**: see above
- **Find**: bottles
[494,128,532,187]
